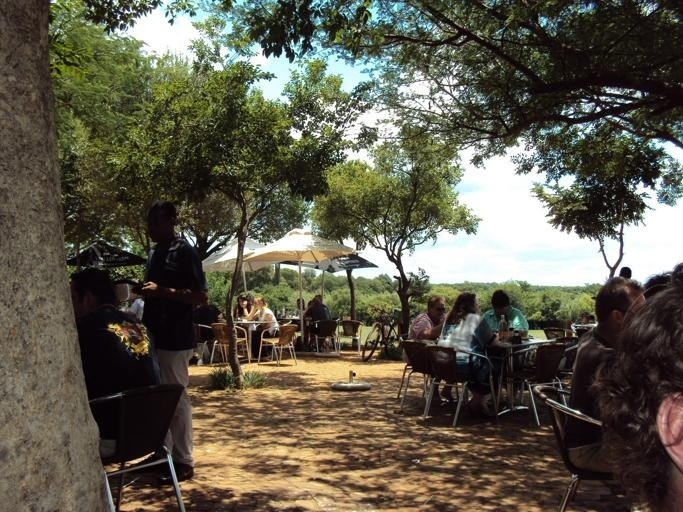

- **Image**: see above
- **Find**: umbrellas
[66,238,148,268]
[181,228,378,343]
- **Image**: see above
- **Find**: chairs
[395,315,613,512]
[195,317,363,369]
[91,382,185,511]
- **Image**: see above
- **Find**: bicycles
[361,302,403,363]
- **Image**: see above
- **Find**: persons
[482,290,529,416]
[189,291,332,365]
[403,297,454,408]
[436,292,497,419]
[118,286,145,323]
[562,262,683,511]
[127,200,205,485]
[71,268,161,466]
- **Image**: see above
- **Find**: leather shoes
[152,463,193,485]
[128,446,173,473]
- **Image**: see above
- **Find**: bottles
[275,304,302,319]
[498,315,528,344]
